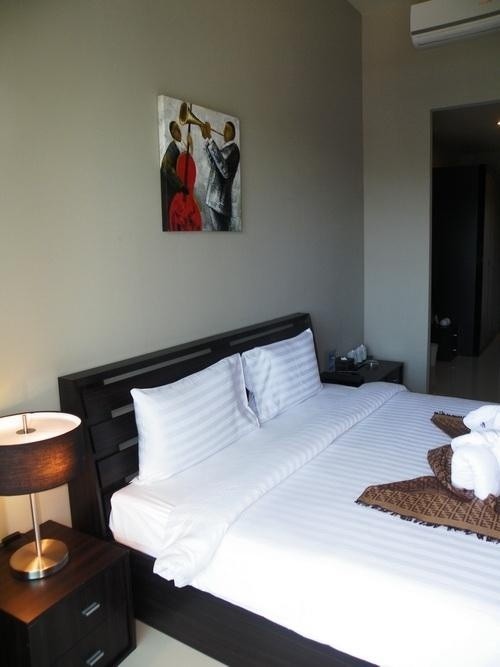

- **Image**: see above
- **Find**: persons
[198,119,241,230]
[161,119,195,230]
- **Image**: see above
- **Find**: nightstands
[325,350,406,386]
[0,520,136,667]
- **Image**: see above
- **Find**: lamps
[0,410,85,578]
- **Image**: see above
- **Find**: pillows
[242,329,323,421]
[131,354,259,482]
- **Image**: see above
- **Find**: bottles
[328,351,337,373]
[347,343,368,366]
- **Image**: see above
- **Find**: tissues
[334,355,355,372]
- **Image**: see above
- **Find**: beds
[58,312,499,667]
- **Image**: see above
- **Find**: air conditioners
[408,1,500,49]
